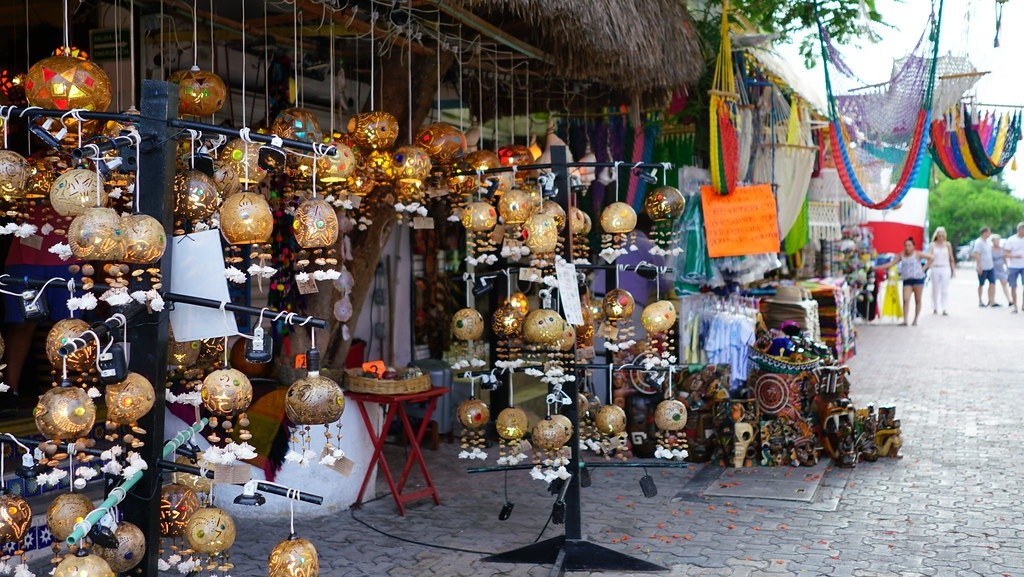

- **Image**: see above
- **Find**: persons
[990,234,1014,306]
[927,226,956,315]
[1003,221,1024,313]
[920,240,928,274]
[974,227,1002,307]
[874,236,935,326]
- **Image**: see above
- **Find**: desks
[343,383,450,517]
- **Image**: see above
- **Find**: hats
[761,284,822,346]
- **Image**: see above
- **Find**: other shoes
[1011,308,1018,313]
[897,322,909,326]
[911,318,918,326]
[987,301,1003,308]
[1021,306,1024,311]
[942,311,949,315]
[978,303,986,307]
[933,309,937,314]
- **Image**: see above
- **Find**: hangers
[700,293,761,320]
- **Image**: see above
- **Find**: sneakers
[1008,301,1013,306]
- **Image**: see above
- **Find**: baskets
[341,364,433,396]
